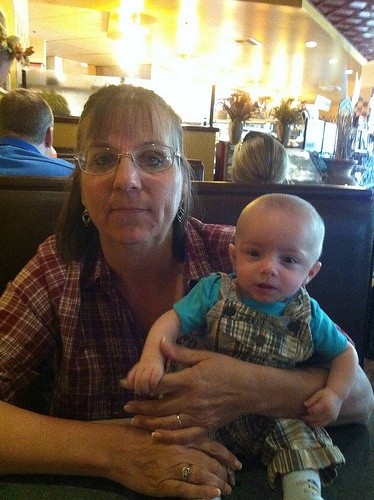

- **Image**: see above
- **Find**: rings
[175,413,186,429]
[181,464,194,483]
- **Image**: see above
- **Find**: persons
[125,193,360,500]
[230,130,289,185]
[0,85,374,500]
[0,88,75,178]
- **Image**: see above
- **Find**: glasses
[74,143,180,176]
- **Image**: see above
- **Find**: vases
[322,158,357,185]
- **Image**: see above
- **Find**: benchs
[0,177,374,467]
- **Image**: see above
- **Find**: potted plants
[269,98,309,148]
[215,90,259,145]
